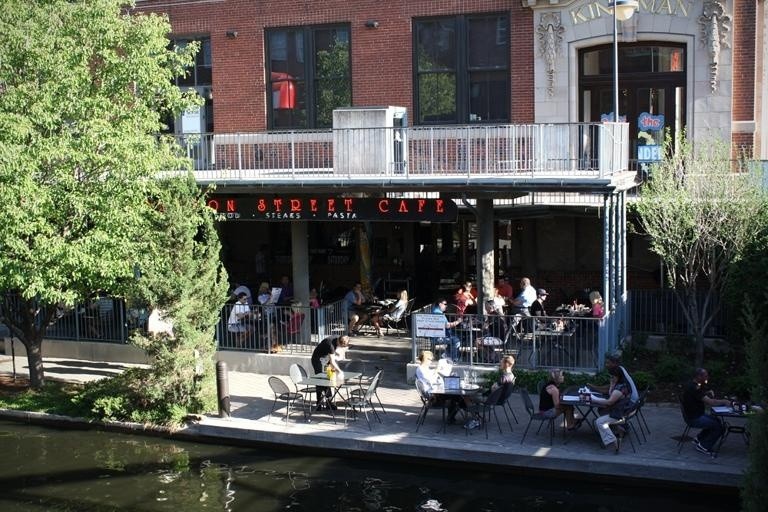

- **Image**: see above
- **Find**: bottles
[327,366,334,380]
[329,368,336,385]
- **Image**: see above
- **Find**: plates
[459,383,480,391]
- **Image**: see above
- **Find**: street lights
[609,0,639,122]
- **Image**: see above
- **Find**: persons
[587,354,640,437]
[579,291,604,337]
[590,367,632,449]
[225,275,322,349]
[86,290,114,340]
[463,355,515,429]
[341,281,365,337]
[431,276,552,366]
[311,334,349,411]
[680,368,732,455]
[540,369,582,431]
[415,350,466,423]
[370,289,408,337]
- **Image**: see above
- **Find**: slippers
[568,419,581,430]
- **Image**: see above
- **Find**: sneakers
[691,437,717,455]
[317,402,336,411]
[615,425,625,451]
[464,416,481,429]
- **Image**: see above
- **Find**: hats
[537,288,548,295]
[605,352,622,363]
